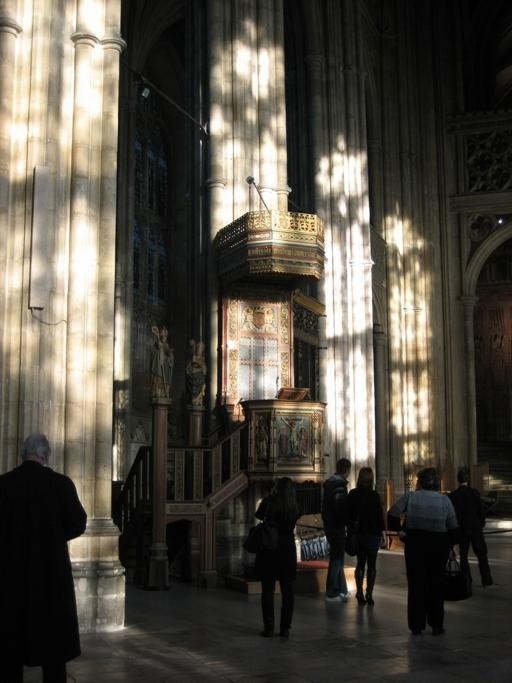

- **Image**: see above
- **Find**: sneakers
[259,629,273,636]
[412,628,447,636]
[325,593,351,603]
[279,630,290,637]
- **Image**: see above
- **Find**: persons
[186,338,207,408]
[344,465,387,607]
[320,457,349,603]
[257,424,270,459]
[151,324,175,396]
[388,465,462,636]
[446,465,493,599]
[245,476,299,638]
[280,416,305,457]
[0,431,88,683]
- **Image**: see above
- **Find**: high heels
[356,592,368,606]
[365,593,375,607]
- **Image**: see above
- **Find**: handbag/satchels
[437,556,472,602]
[242,516,278,553]
[346,531,361,556]
